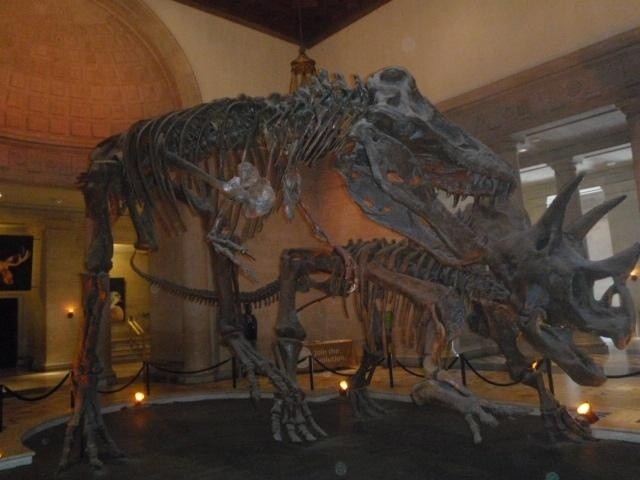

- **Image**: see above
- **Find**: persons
[242,304,259,376]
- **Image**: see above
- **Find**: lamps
[339,380,349,395]
[135,392,145,407]
[67,308,75,318]
[577,403,598,424]
[288,6,317,95]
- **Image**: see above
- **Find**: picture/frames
[0,224,42,300]
